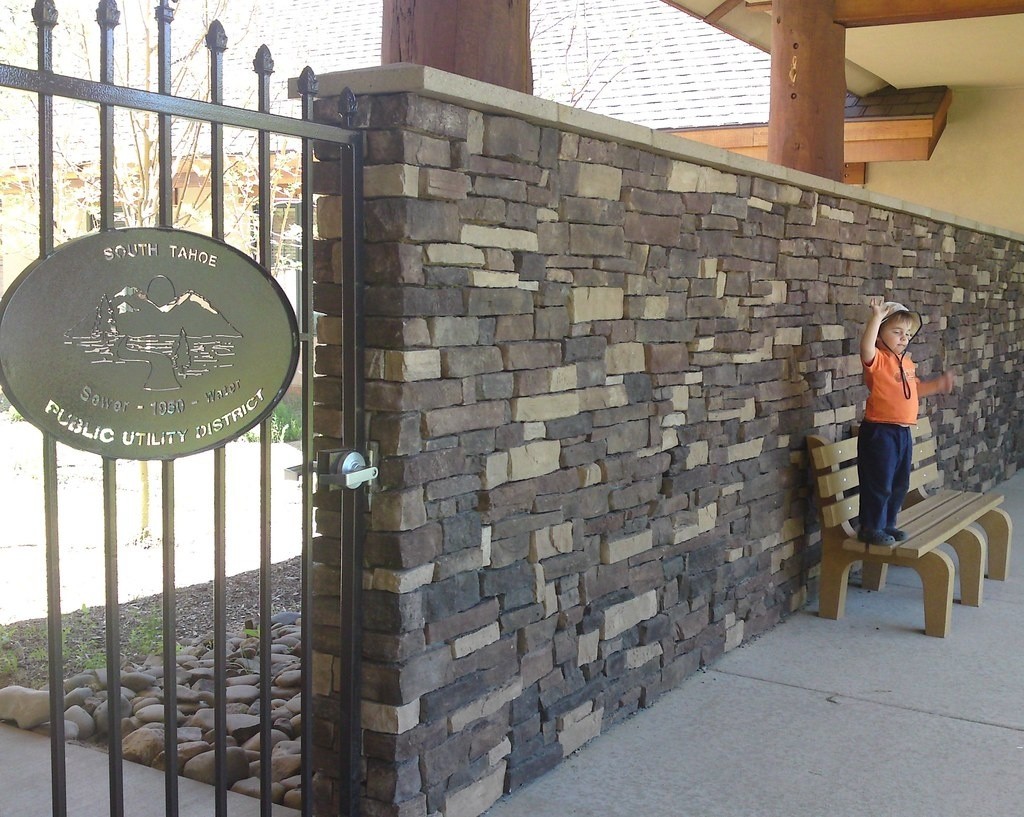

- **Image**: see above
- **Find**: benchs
[808,398,1012,637]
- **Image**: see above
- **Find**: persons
[857,298,955,545]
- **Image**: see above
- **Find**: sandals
[857,528,896,544]
[887,528,908,541]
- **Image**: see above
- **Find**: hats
[867,302,922,347]
